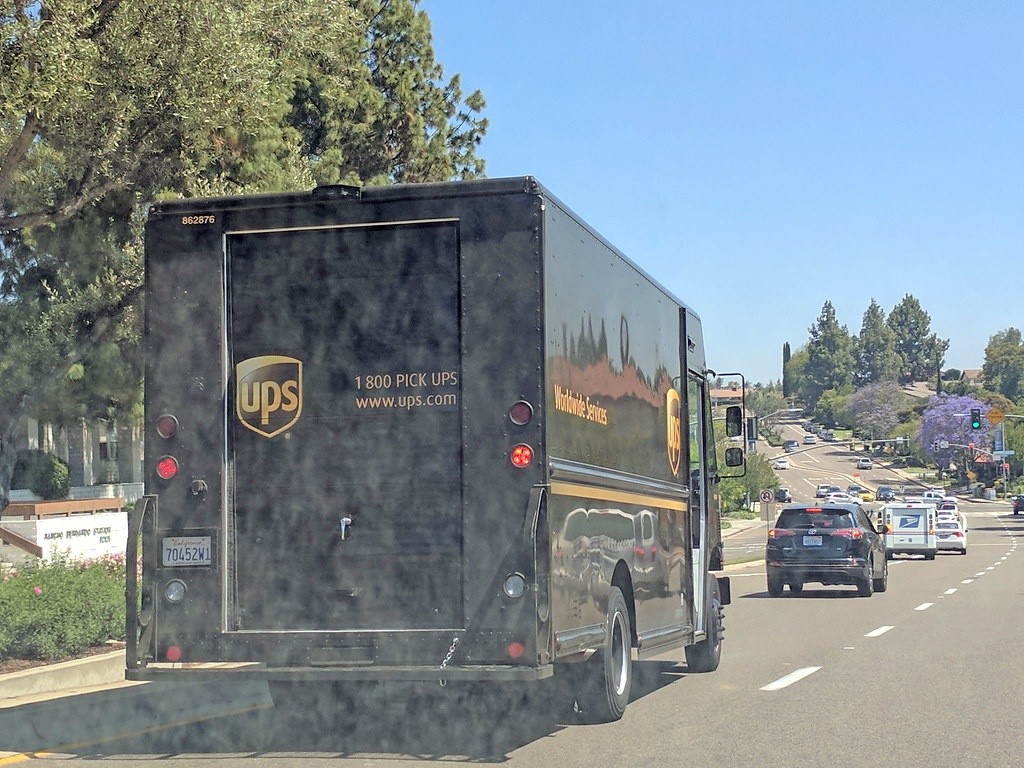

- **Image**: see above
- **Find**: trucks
[126,177,760,724]
[854,500,938,560]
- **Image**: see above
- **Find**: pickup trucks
[902,492,944,503]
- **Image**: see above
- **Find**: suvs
[824,493,863,506]
[846,485,862,497]
[767,503,889,596]
[778,488,791,503]
[877,487,896,502]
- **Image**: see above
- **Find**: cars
[934,521,968,556]
[815,484,831,497]
[730,436,739,441]
[856,457,872,469]
[802,421,835,442]
[936,469,956,479]
[776,460,789,470]
[825,486,842,492]
[552,505,668,595]
[937,497,960,522]
[803,435,816,445]
[784,439,799,452]
[1012,494,1024,515]
[857,489,874,501]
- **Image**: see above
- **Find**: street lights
[746,408,803,509]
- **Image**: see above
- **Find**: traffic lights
[934,442,939,452]
[999,464,1003,474]
[969,443,974,456]
[1005,464,1009,469]
[971,409,981,429]
